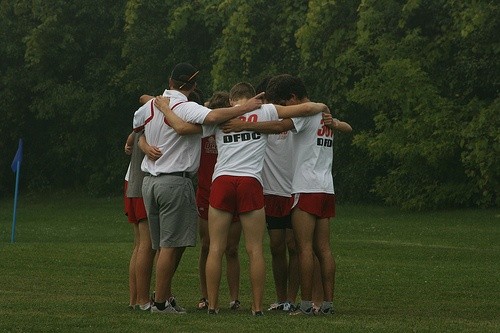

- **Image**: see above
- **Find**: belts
[144,171,191,177]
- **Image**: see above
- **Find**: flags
[10,139,23,172]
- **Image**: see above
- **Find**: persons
[220,74,353,316]
[124,81,330,318]
[133,62,264,317]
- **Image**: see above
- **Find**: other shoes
[196,298,207,310]
[173,304,186,312]
[231,302,240,310]
[134,303,151,311]
[268,302,294,311]
[318,305,334,315]
[151,302,179,313]
[290,305,316,315]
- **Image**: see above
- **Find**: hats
[171,62,199,84]
